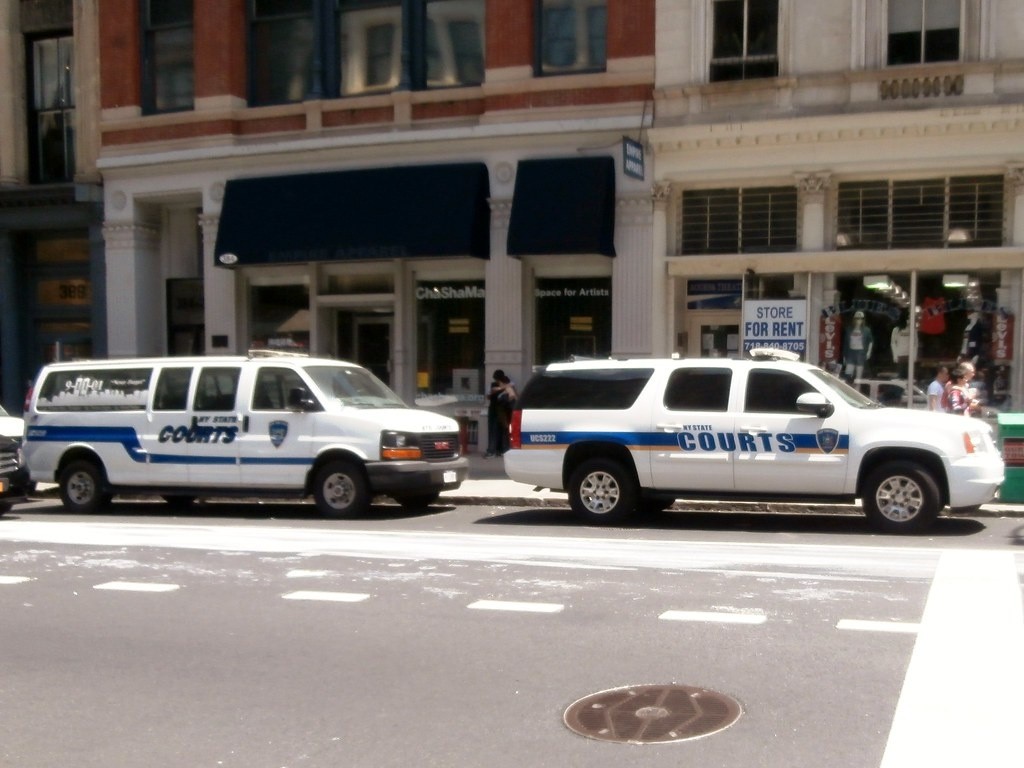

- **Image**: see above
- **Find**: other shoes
[484,451,497,460]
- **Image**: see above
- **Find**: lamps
[943,273,968,287]
[864,276,911,307]
[218,238,239,264]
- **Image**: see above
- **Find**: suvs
[844,374,928,409]
[500,344,1006,529]
[23,349,469,516]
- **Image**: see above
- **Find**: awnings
[214,155,492,269]
[506,155,617,259]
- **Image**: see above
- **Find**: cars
[0,436,33,516]
[1,404,23,439]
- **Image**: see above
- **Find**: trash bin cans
[453,416,468,456]
[996,413,1024,503]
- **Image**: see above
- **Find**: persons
[482,369,520,460]
[956,309,983,365]
[941,362,982,417]
[24,379,34,412]
[843,311,873,393]
[926,365,949,411]
[891,319,918,400]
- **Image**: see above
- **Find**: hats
[853,311,865,318]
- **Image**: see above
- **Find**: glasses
[855,317,864,320]
